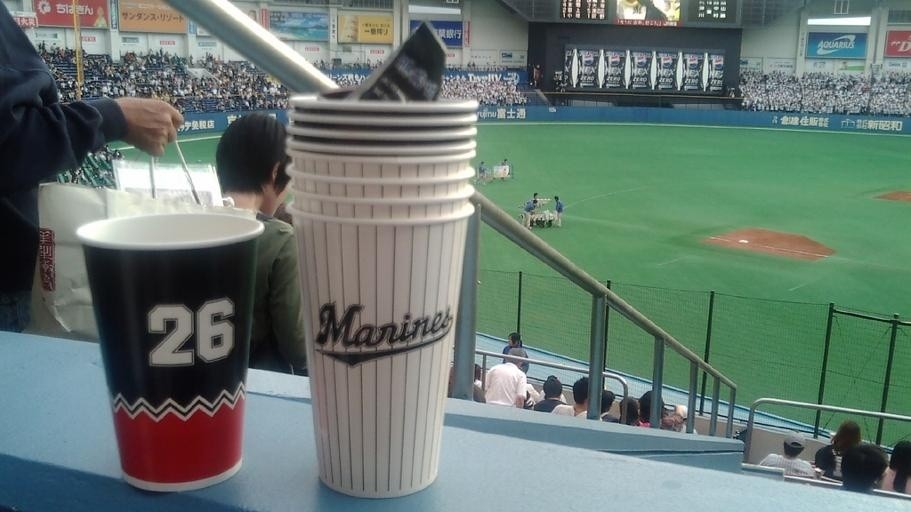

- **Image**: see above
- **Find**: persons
[437,60,569,106]
[38,40,290,115]
[213,111,307,373]
[522,200,536,230]
[473,160,487,187]
[449,332,697,434]
[307,56,385,88]
[498,158,508,182]
[737,67,910,118]
[762,419,910,499]
[532,192,541,214]
[553,196,563,228]
[0,0,185,334]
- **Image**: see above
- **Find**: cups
[76,209,267,495]
[285,94,476,498]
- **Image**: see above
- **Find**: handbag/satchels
[31,137,256,343]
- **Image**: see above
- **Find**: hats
[676,405,688,419]
[785,432,805,448]
